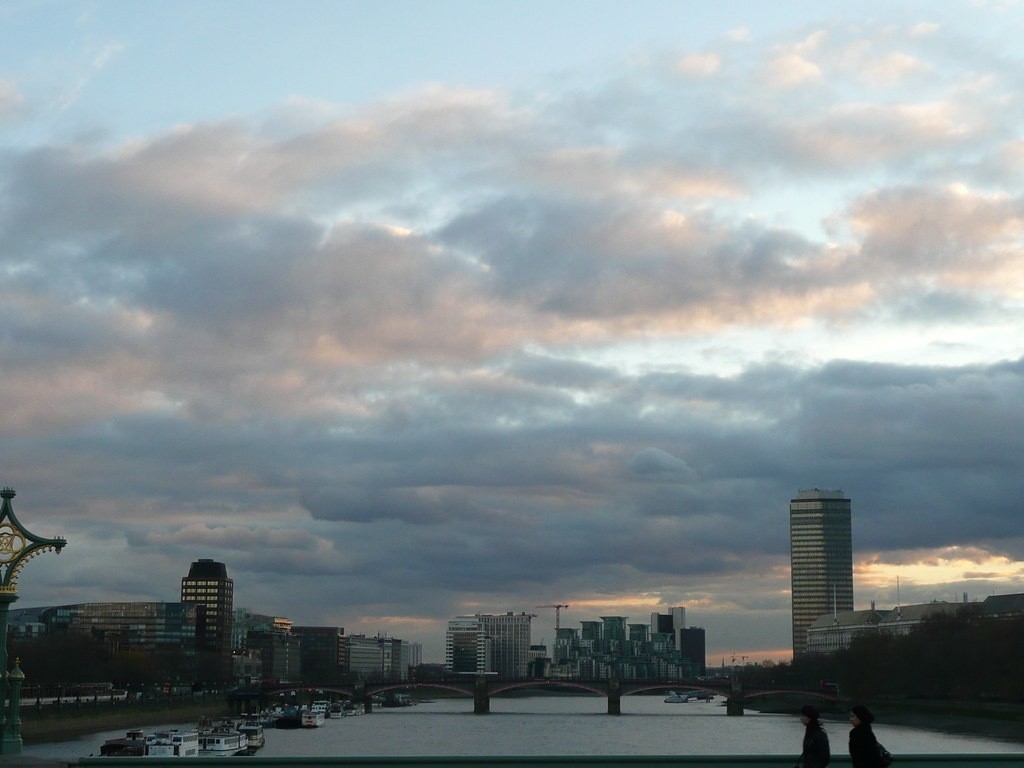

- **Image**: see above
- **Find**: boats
[382,692,417,707]
[697,694,715,699]
[664,690,690,703]
[98,700,366,758]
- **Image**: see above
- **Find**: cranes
[731,655,749,666]
[533,604,570,626]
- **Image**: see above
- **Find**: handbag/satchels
[875,741,893,768]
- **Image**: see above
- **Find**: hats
[853,705,874,724]
[802,704,819,721]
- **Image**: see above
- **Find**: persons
[847,705,895,768]
[793,704,830,768]
